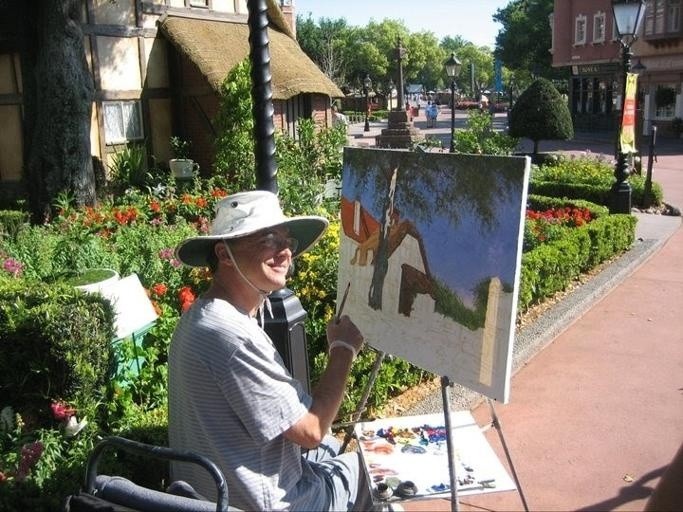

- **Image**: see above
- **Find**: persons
[424,101,430,127]
[429,103,438,128]
[486,100,495,117]
[164,191,401,511]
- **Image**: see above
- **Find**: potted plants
[167,132,200,176]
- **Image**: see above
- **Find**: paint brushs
[336,282,350,324]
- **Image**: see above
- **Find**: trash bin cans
[427,111,437,128]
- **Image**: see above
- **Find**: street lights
[629,56,646,148]
[443,52,463,152]
[363,74,372,131]
[507,74,516,110]
[605,1,649,215]
[404,82,411,104]
[387,78,393,112]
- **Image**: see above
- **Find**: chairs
[58,427,242,512]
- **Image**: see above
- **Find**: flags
[618,72,639,155]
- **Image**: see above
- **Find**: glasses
[235,231,300,257]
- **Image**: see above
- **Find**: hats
[176,188,330,266]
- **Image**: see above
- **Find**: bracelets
[328,340,358,362]
